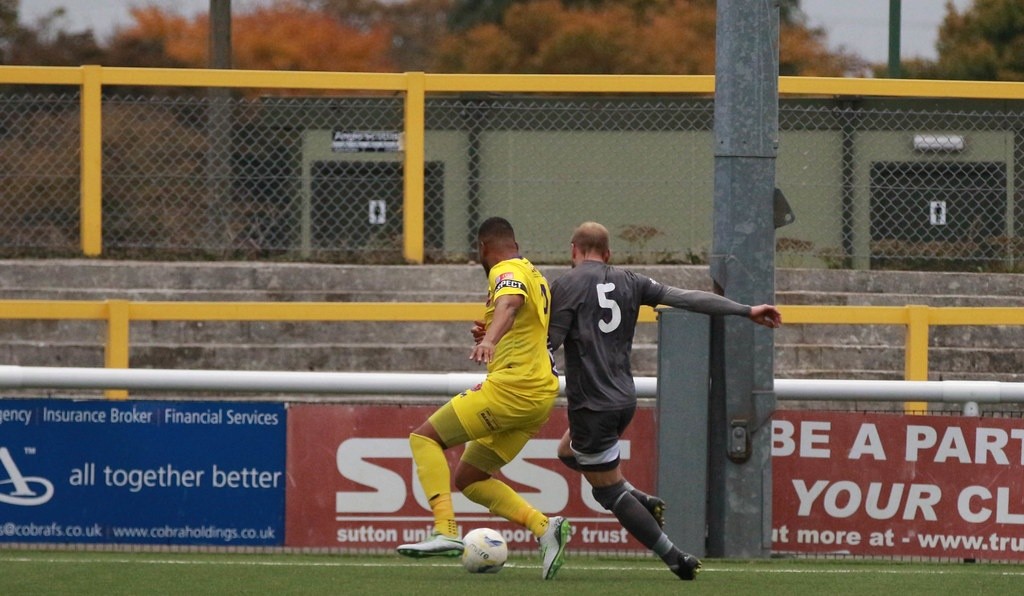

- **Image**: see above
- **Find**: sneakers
[671,551,702,580]
[536,516,571,580]
[641,495,666,530]
[396,531,465,559]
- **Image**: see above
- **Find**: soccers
[460,528,508,574]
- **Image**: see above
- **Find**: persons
[397,216,570,581]
[548,222,782,579]
